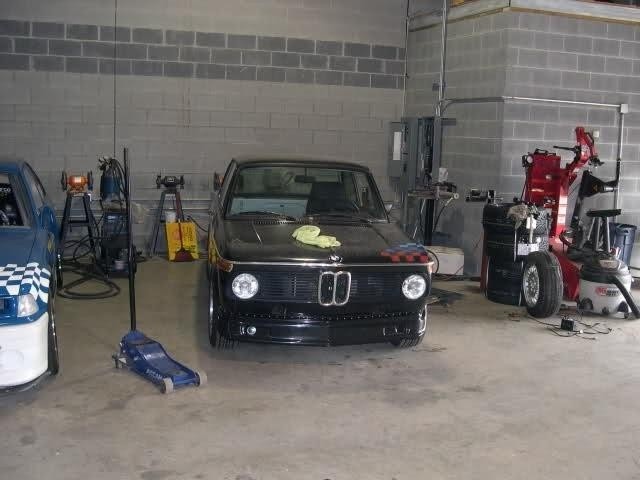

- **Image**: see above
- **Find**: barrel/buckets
[604,222,636,269]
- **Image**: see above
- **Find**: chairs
[0,182,18,225]
[305,180,348,214]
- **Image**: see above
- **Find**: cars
[205,156,433,351]
[0,156,62,395]
